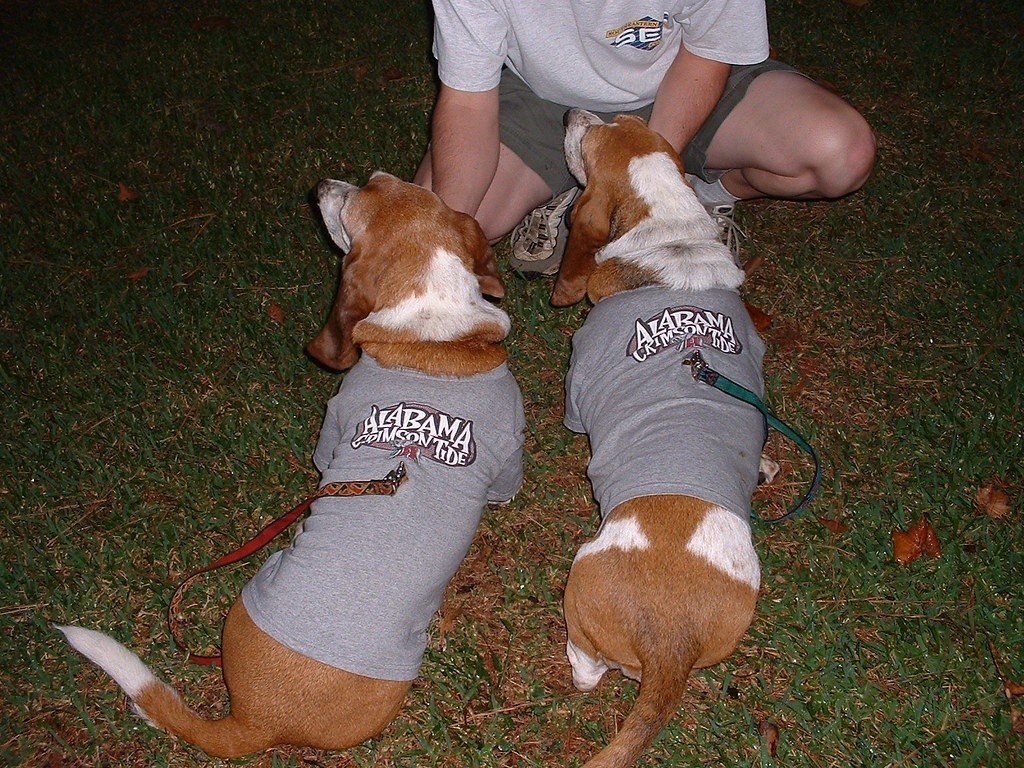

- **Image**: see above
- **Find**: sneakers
[682,171,746,248]
[510,187,583,278]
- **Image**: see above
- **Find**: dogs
[551,107,779,768]
[55,170,525,756]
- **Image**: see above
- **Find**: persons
[413,0,875,282]
